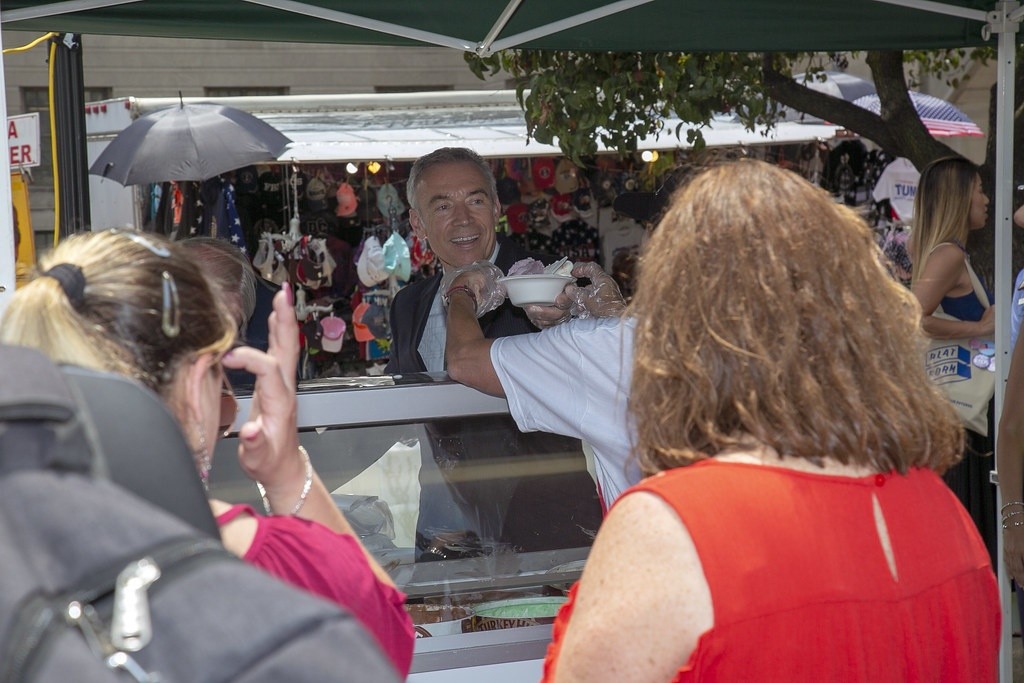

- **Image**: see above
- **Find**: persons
[880,156,1024,672]
[1,224,414,682]
[157,232,257,322]
[441,261,645,519]
[382,147,608,547]
[538,151,1003,683]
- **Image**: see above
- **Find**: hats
[237,166,411,353]
[489,158,594,233]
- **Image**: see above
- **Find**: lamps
[346,162,360,174]
[367,161,381,173]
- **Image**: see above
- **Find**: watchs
[441,286,479,316]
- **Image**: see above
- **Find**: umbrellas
[85,88,294,189]
[734,71,982,140]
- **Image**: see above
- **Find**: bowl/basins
[497,275,575,307]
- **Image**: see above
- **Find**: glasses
[190,352,239,439]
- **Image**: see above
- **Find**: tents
[117,1,1024,683]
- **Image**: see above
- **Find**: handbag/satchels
[917,242,995,437]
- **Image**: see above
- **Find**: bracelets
[1002,510,1024,521]
[1001,501,1023,513]
[1002,521,1023,528]
[257,443,314,519]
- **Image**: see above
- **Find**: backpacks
[0,347,403,683]
